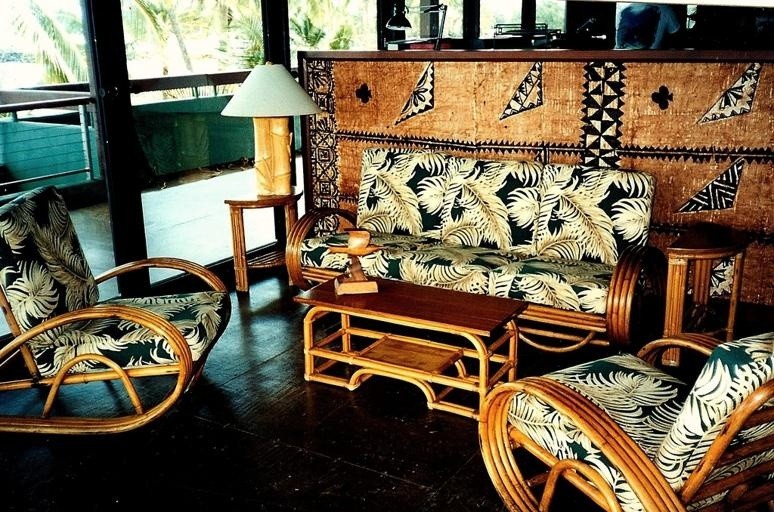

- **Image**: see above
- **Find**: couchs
[285,146,671,356]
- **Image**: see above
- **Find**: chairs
[0,193,231,436]
[476,332,774,512]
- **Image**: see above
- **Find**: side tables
[224,186,303,292]
[660,220,750,367]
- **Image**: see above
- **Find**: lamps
[385,2,447,52]
[220,61,323,196]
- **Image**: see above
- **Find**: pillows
[356,147,451,240]
[0,173,99,351]
[440,154,544,255]
[652,332,774,512]
[537,162,656,267]
